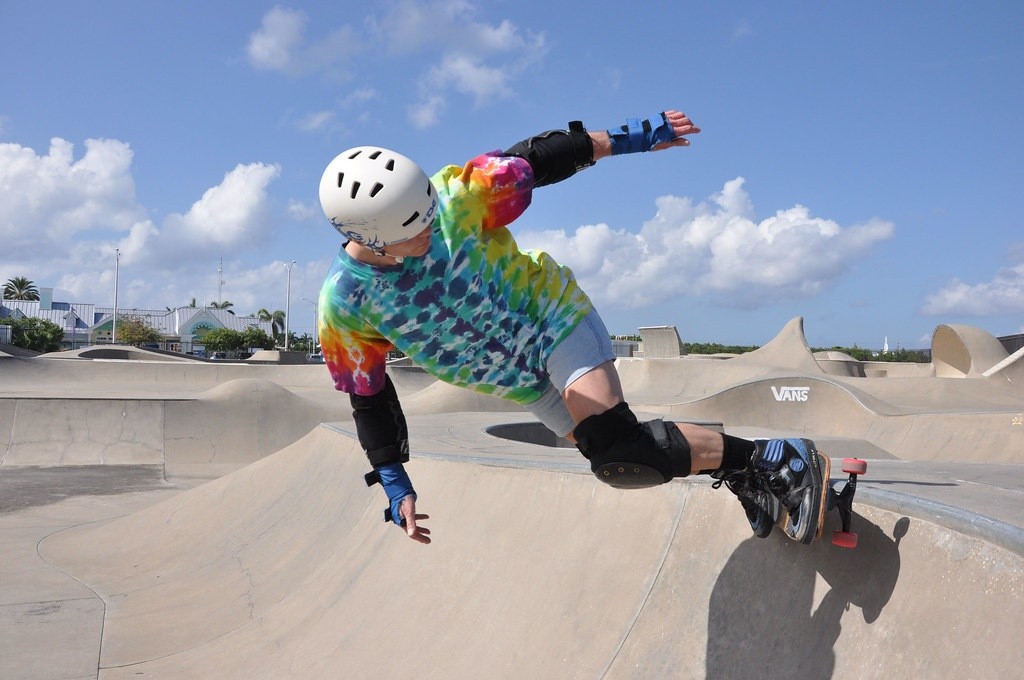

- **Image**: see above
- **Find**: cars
[238,353,252,359]
[213,352,225,359]
[305,353,323,362]
[192,350,207,358]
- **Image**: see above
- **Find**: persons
[319,108,823,543]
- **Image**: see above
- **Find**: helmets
[319,146,440,250]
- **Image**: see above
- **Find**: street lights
[300,298,317,355]
[112,248,122,344]
[283,260,297,352]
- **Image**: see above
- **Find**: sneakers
[709,438,821,545]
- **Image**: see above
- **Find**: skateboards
[774,438,867,551]
